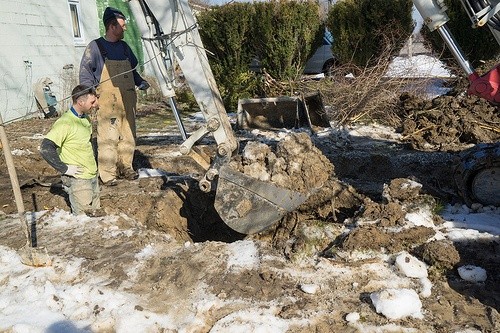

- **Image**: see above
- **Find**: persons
[40,85,101,214]
[79,7,150,186]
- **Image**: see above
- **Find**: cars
[302,27,340,73]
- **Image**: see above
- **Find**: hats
[103,6,126,26]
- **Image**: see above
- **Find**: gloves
[137,81,150,90]
[64,164,84,179]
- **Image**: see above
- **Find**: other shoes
[104,178,121,186]
[119,167,139,180]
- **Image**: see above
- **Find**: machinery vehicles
[122,0,500,235]
